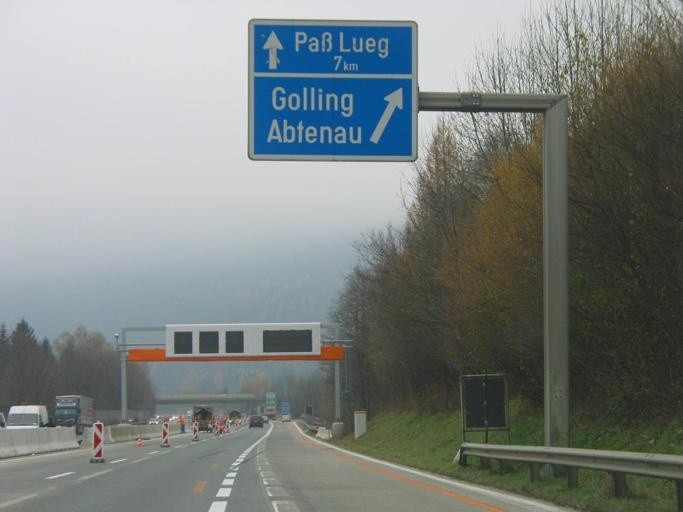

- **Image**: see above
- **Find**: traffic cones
[133,433,143,448]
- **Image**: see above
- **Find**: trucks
[54,394,95,436]
[190,405,215,432]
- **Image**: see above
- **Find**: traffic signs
[247,13,424,168]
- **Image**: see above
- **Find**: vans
[6,404,50,431]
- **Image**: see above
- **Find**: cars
[249,415,278,428]
[0,412,8,430]
[148,413,183,425]
[281,414,291,422]
